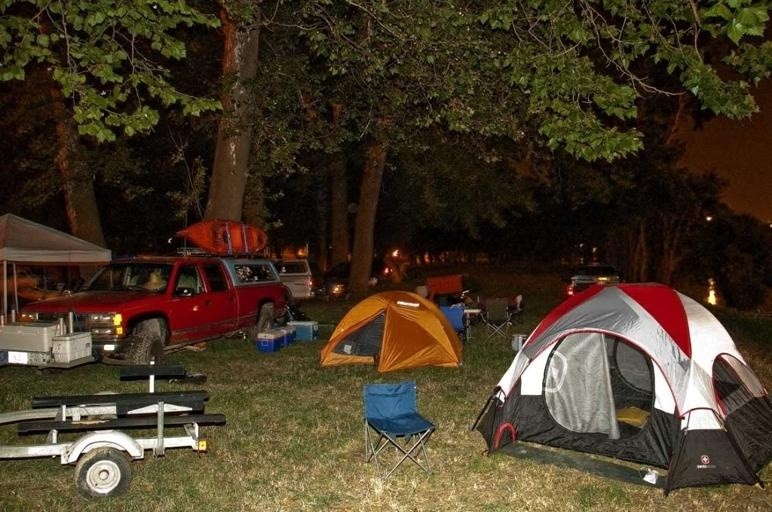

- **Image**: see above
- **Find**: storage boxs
[49,331,92,363]
[255,328,284,354]
[289,320,319,344]
[276,323,298,345]
[0,320,63,353]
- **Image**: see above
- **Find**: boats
[175,219,268,258]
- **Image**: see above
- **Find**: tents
[314,288,466,373]
[477,280,772,500]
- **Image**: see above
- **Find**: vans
[274,258,322,306]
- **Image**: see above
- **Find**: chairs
[480,296,512,347]
[357,380,435,473]
[439,303,468,346]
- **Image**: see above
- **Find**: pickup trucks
[18,255,294,366]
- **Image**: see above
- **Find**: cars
[0,266,40,295]
[315,261,385,300]
[564,263,627,299]
